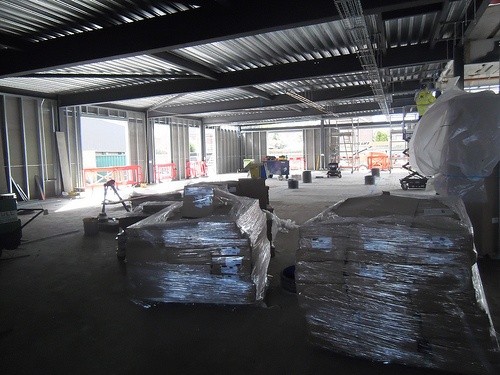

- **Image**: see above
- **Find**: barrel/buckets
[289,179,299,190]
[1,219,22,250]
[365,175,375,186]
[82,217,99,235]
[302,170,312,183]
[371,168,380,179]
